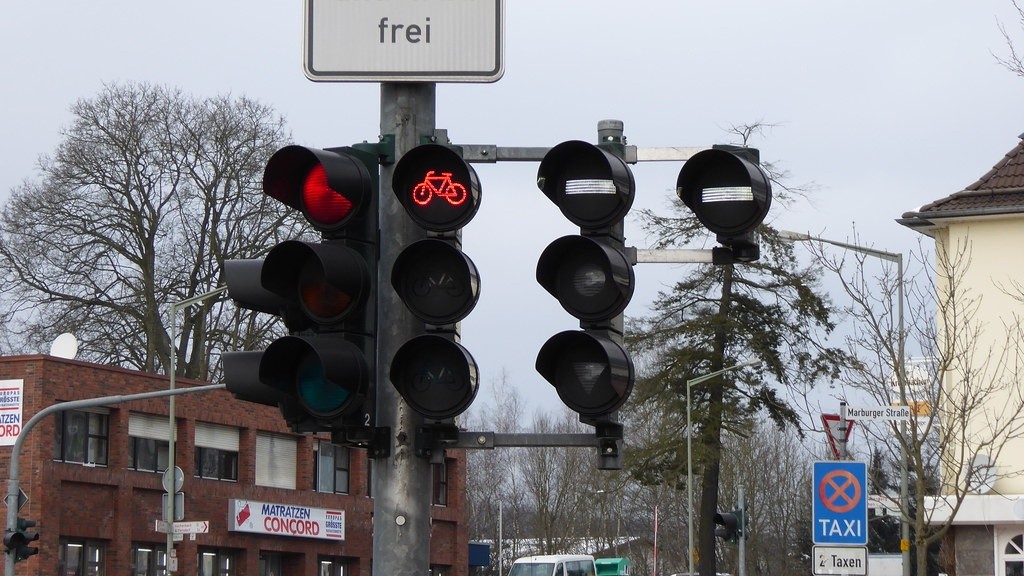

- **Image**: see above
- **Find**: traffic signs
[155,519,209,534]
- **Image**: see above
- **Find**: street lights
[778,229,910,576]
[687,359,763,576]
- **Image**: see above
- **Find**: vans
[507,554,597,576]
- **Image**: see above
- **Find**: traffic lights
[536,141,635,420]
[257,144,380,432]
[713,510,739,541]
[222,257,280,407]
[3,531,25,549]
[17,516,39,559]
[388,144,482,427]
[676,144,773,247]
